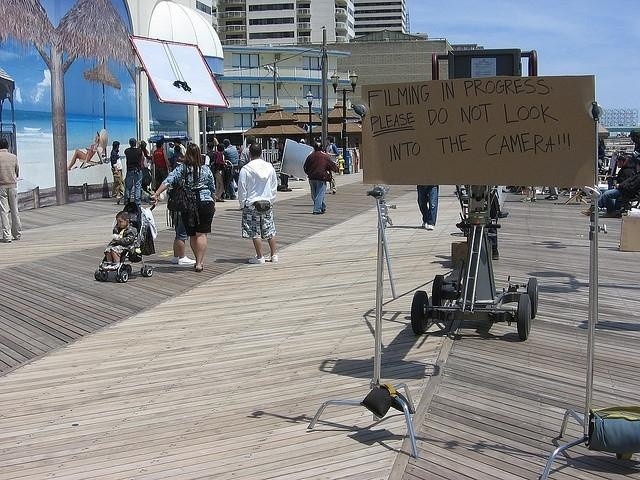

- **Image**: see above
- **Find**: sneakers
[170,255,197,266]
[270,254,279,264]
[544,195,556,200]
[102,264,120,271]
[601,209,618,217]
[422,222,434,230]
[519,196,532,203]
[247,253,266,265]
[312,208,326,215]
[216,198,225,202]
[579,209,592,216]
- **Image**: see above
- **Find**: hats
[614,156,626,161]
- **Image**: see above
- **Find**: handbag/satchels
[166,177,187,211]
[251,199,272,212]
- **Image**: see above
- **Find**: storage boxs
[618,211,640,252]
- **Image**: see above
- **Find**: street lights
[329,66,360,179]
[304,90,315,153]
[249,97,260,138]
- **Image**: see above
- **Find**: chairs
[78,144,103,170]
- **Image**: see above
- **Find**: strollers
[224,159,239,201]
[133,166,158,202]
[93,190,164,286]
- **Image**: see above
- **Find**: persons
[0,138,22,242]
[238,142,278,264]
[150,144,216,272]
[296,137,339,215]
[520,129,640,218]
[277,141,288,187]
[168,160,197,266]
[110,138,250,207]
[67,136,100,170]
[415,185,439,230]
[104,211,135,270]
